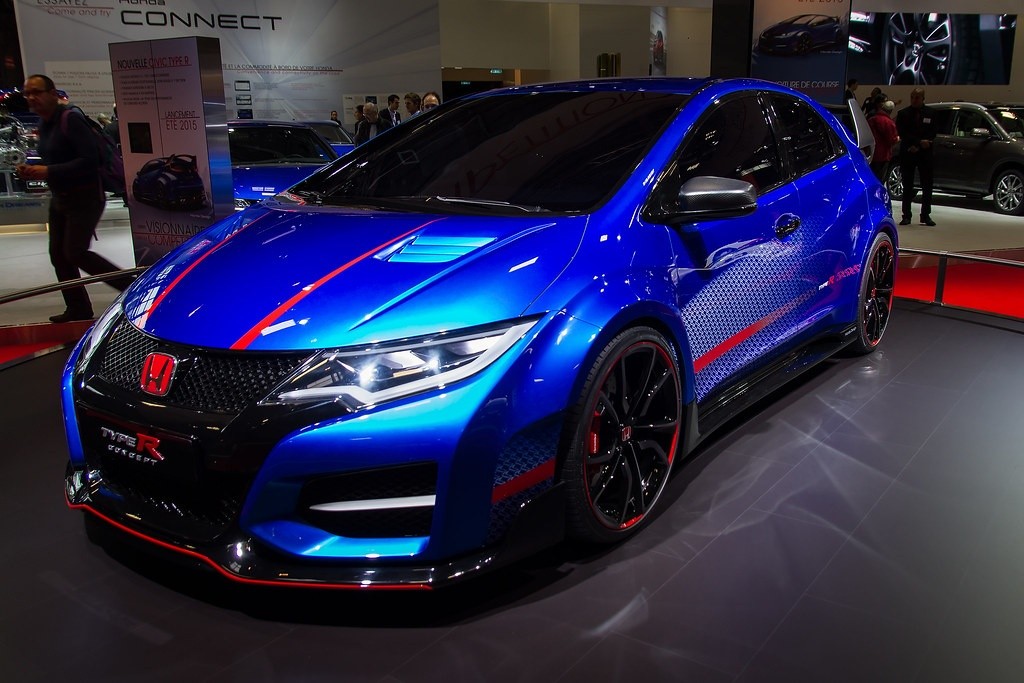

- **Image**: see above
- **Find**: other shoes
[920,218,936,226]
[49,309,94,323]
[109,194,120,197]
[899,218,911,226]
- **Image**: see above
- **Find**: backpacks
[59,110,126,195]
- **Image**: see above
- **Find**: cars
[884,98,1024,216]
[755,13,843,59]
[132,151,206,212]
[299,120,357,164]
[224,120,340,215]
[55,77,899,595]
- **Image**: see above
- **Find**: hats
[883,101,895,110]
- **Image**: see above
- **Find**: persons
[870,93,888,118]
[861,87,881,116]
[110,114,118,121]
[404,92,422,125]
[96,112,122,197]
[896,87,937,227]
[354,103,385,147]
[422,91,441,113]
[24,74,139,323]
[354,104,365,135]
[379,94,402,131]
[106,102,128,209]
[845,78,858,102]
[330,111,342,127]
[868,100,900,186]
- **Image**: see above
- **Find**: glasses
[21,88,49,98]
[363,113,372,117]
